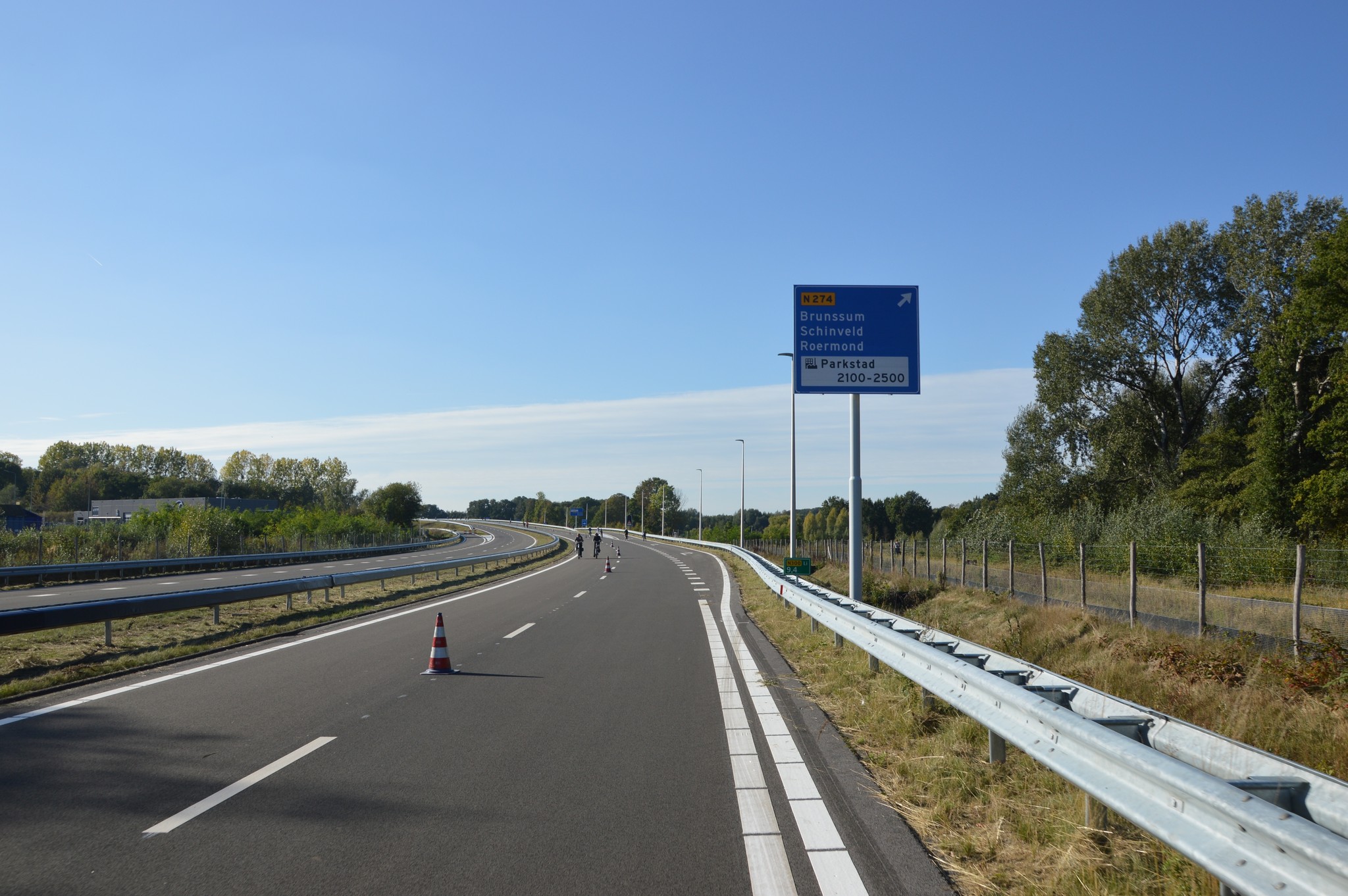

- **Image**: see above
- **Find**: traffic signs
[582,519,587,526]
[793,284,921,395]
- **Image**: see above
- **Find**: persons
[592,532,601,557]
[572,524,574,529]
[523,521,529,528]
[509,520,511,524]
[596,526,603,537]
[623,528,628,539]
[643,531,647,542]
[588,526,592,536]
[574,533,584,558]
[420,516,486,521]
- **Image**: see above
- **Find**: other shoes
[580,555,582,557]
[576,551,577,553]
[593,554,595,557]
[598,549,600,553]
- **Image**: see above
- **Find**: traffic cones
[615,546,621,556]
[611,540,614,547]
[569,531,571,534]
[419,612,460,675]
[552,528,554,531]
[535,525,536,528]
[584,533,587,538]
[603,556,612,573]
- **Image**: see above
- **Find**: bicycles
[592,541,602,559]
[574,541,584,559]
[623,533,628,541]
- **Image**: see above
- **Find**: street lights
[778,352,796,557]
[736,439,744,548]
[696,469,702,541]
